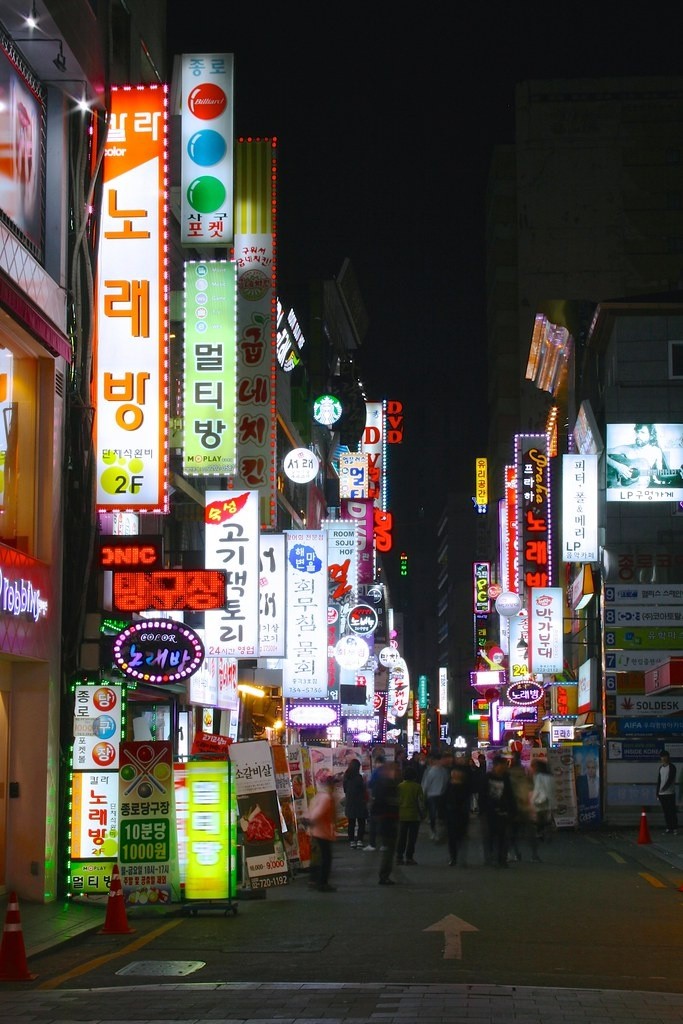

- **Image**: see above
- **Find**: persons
[296,768,339,894]
[653,749,679,841]
[341,747,555,884]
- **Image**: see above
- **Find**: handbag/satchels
[537,809,552,826]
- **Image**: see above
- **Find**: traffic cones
[636,806,652,845]
[94,862,137,934]
[0,888,40,982]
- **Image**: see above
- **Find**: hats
[322,775,340,783]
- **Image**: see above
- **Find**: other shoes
[362,845,375,851]
[318,885,337,892]
[357,841,363,849]
[431,831,439,841]
[449,857,457,866]
[662,829,672,835]
[350,841,355,848]
[397,861,404,865]
[379,845,388,851]
[673,829,679,837]
[379,878,394,884]
[406,858,417,864]
